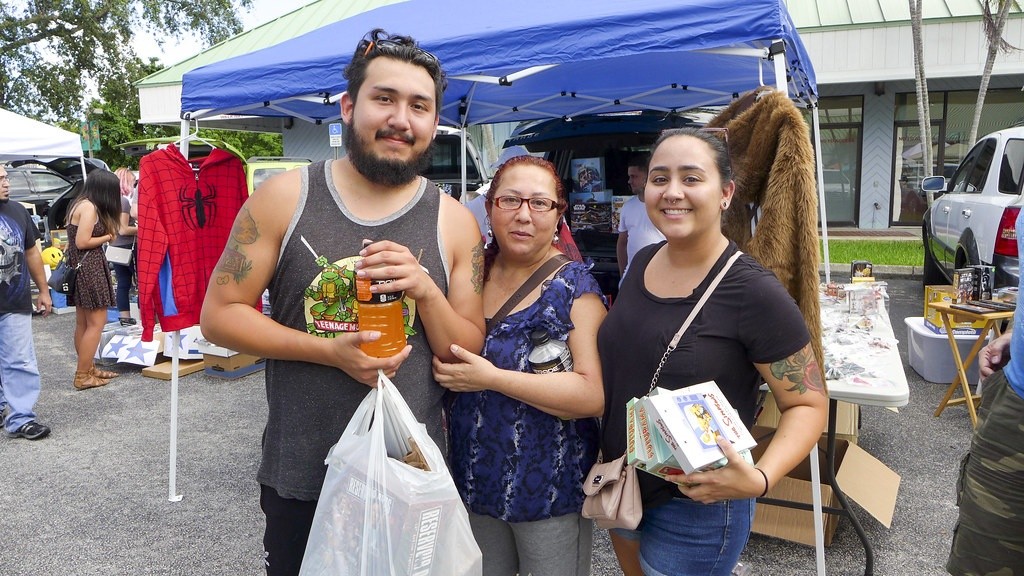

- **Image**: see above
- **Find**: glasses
[0,177,10,182]
[654,127,731,162]
[364,39,439,66]
[490,196,562,212]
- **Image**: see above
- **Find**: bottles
[528,329,574,420]
[354,241,406,358]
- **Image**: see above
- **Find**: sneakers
[0,410,6,427]
[9,421,50,439]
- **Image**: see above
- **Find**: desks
[800,283,909,576]
[928,299,1016,431]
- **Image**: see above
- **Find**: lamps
[874,82,885,95]
[284,117,293,129]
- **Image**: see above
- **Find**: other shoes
[118,317,136,326]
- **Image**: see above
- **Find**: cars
[824,168,855,207]
[112,136,315,199]
[418,123,495,207]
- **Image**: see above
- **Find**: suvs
[6,168,75,219]
[920,126,1024,292]
[900,163,959,215]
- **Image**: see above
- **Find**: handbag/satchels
[47,260,78,294]
[105,245,132,266]
[297,371,483,576]
[582,453,643,530]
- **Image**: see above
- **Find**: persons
[108,168,139,319]
[200,30,486,576]
[945,204,1024,576]
[0,166,51,440]
[66,169,122,389]
[616,163,667,289]
[595,129,829,576]
[436,156,609,576]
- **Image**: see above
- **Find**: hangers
[174,112,217,151]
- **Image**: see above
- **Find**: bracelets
[755,468,768,498]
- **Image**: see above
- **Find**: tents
[135,0,826,576]
[0,109,87,186]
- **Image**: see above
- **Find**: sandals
[74,370,110,390]
[89,363,119,378]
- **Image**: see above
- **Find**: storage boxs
[903,316,996,385]
[143,322,267,381]
[924,264,994,336]
[851,260,876,283]
[747,391,900,548]
[569,156,633,234]
